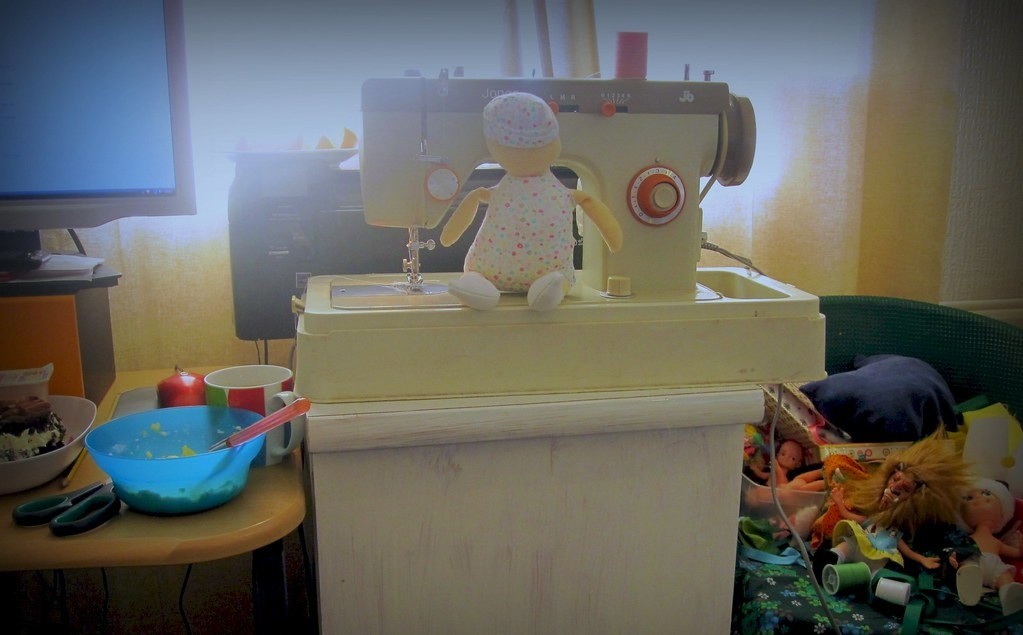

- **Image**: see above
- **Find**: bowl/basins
[83,405,267,515]
[0,394,97,495]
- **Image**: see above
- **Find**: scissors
[12,478,122,537]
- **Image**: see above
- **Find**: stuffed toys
[439,91,621,310]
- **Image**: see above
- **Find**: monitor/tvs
[0,0,198,276]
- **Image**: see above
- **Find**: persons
[949,479,1023,617]
[750,440,876,547]
[813,427,972,589]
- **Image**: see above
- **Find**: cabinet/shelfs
[305,380,763,635]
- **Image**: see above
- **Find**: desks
[0,368,306,635]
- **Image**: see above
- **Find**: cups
[203,365,305,468]
